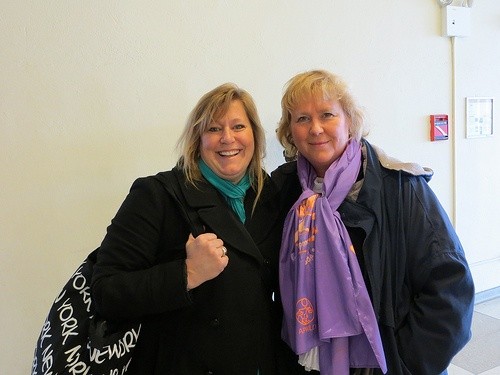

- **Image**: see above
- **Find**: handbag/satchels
[29,248,147,375]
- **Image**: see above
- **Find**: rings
[220,247,226,258]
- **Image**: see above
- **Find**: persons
[272,70,475,374]
[90,81,281,374]
